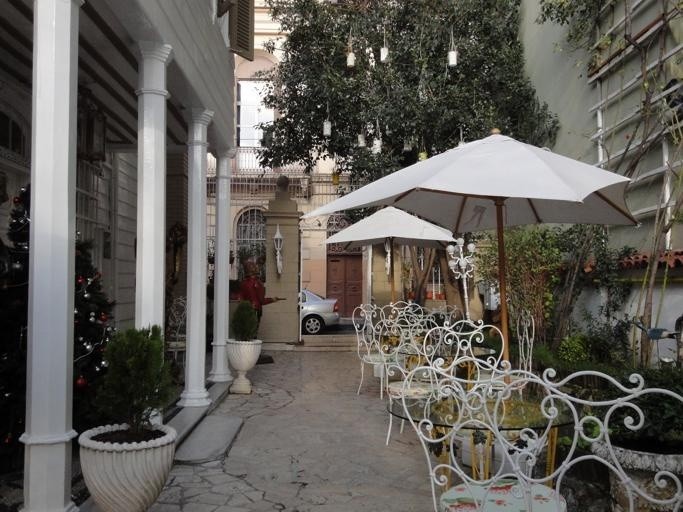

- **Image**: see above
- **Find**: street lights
[446,237,476,320]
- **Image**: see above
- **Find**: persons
[239,262,278,339]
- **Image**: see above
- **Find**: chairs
[402,356,569,512]
[350,296,566,476]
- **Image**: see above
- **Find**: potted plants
[75,323,186,512]
[590,322,683,512]
[226,300,263,394]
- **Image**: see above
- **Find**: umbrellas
[299,129,641,385]
[318,206,457,305]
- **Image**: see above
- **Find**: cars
[301,289,339,335]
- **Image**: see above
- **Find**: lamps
[273,222,285,274]
